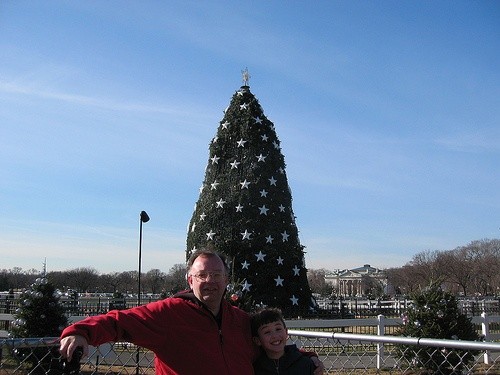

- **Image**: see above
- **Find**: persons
[58,249,325,375]
[250,309,318,375]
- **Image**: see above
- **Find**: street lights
[135,211,151,375]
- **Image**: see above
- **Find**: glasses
[190,271,227,282]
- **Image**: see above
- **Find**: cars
[471,292,481,296]
[459,292,464,296]
